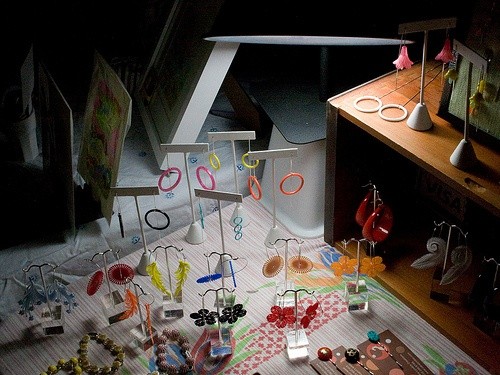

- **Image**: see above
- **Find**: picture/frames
[436,0,500,154]
[135,0,250,173]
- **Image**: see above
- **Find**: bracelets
[155,328,195,375]
[38,332,124,375]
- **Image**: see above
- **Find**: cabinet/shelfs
[323,52,500,375]
